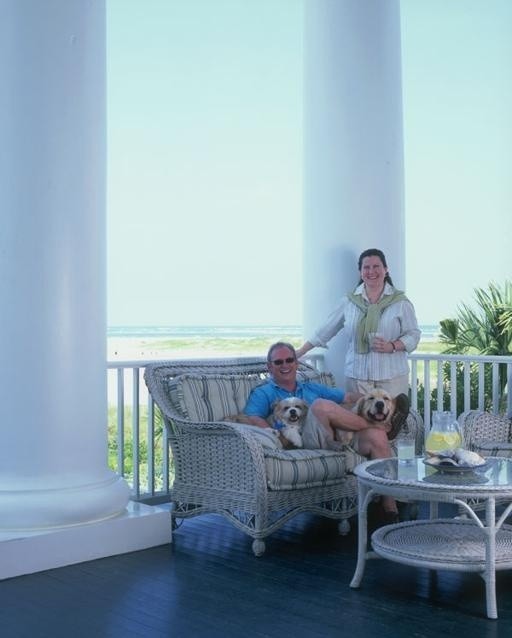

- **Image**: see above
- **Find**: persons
[291,247,422,441]
[244,343,411,527]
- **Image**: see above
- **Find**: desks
[348,454,512,619]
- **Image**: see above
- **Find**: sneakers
[387,393,409,440]
[375,501,399,523]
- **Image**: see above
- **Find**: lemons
[432,432,459,444]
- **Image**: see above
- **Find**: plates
[422,473,489,485]
[423,460,486,472]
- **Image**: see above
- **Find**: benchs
[143,357,424,557]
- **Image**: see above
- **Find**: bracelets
[389,342,397,353]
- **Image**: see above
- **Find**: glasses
[270,358,294,365]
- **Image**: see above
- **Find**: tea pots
[425,411,463,457]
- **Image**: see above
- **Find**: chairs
[458,409,512,520]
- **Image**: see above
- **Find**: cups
[399,461,419,484]
[367,332,384,350]
[397,436,415,467]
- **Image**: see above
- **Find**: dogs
[335,388,397,445]
[224,397,309,450]
